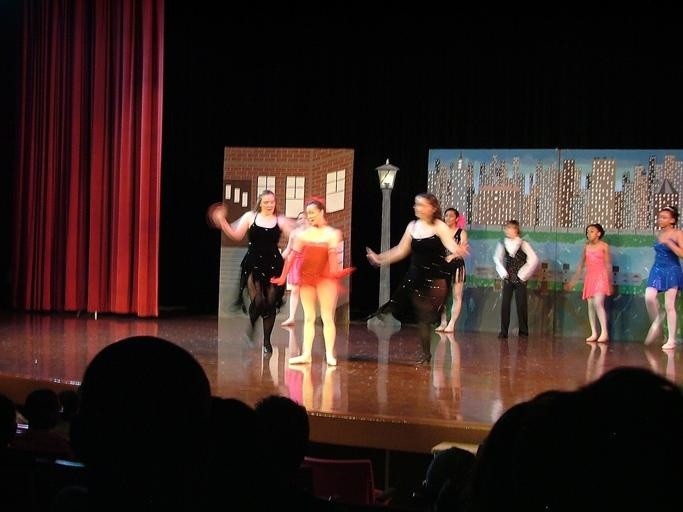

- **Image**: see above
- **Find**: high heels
[414,351,433,366]
[262,341,273,354]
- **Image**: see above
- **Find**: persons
[644,205,683,350]
[564,223,615,342]
[435,208,469,332]
[270,197,357,367]
[1,335,318,511]
[366,192,461,366]
[209,189,299,353]
[281,211,309,327]
[493,219,539,339]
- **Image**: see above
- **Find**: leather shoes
[518,333,529,342]
[498,332,508,340]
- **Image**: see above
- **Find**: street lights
[367,158,401,328]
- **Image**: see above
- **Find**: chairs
[303,455,384,506]
